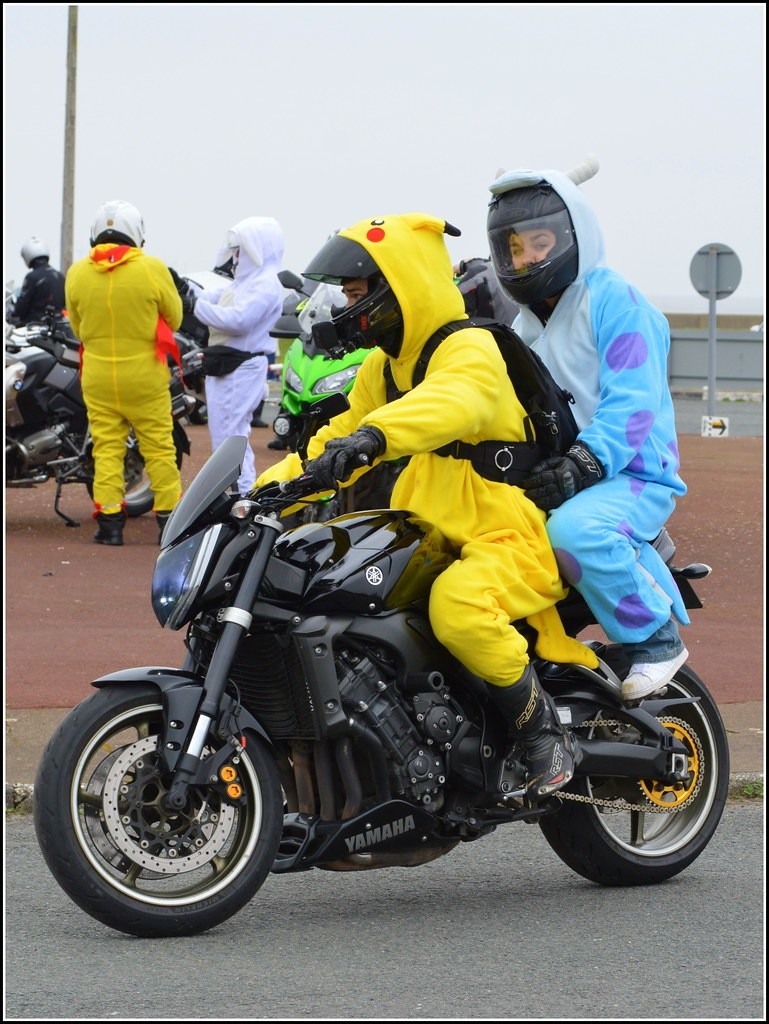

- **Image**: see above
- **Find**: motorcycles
[273,258,508,451]
[31,436,730,939]
[2,293,210,528]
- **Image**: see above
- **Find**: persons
[64,199,182,544]
[481,152,693,702]
[5,237,68,327]
[266,277,348,451]
[181,215,287,502]
[241,211,604,802]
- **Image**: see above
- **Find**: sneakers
[92,511,126,546]
[155,512,173,546]
[621,644,690,700]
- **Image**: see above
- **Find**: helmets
[486,188,578,305]
[89,200,146,249]
[301,236,402,360]
[20,235,50,269]
[453,257,499,316]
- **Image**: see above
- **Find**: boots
[482,664,584,804]
[250,399,269,428]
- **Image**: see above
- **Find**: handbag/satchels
[201,344,265,377]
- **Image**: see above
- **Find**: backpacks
[382,317,581,491]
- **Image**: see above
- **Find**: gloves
[523,440,605,511]
[182,288,199,315]
[304,424,388,489]
